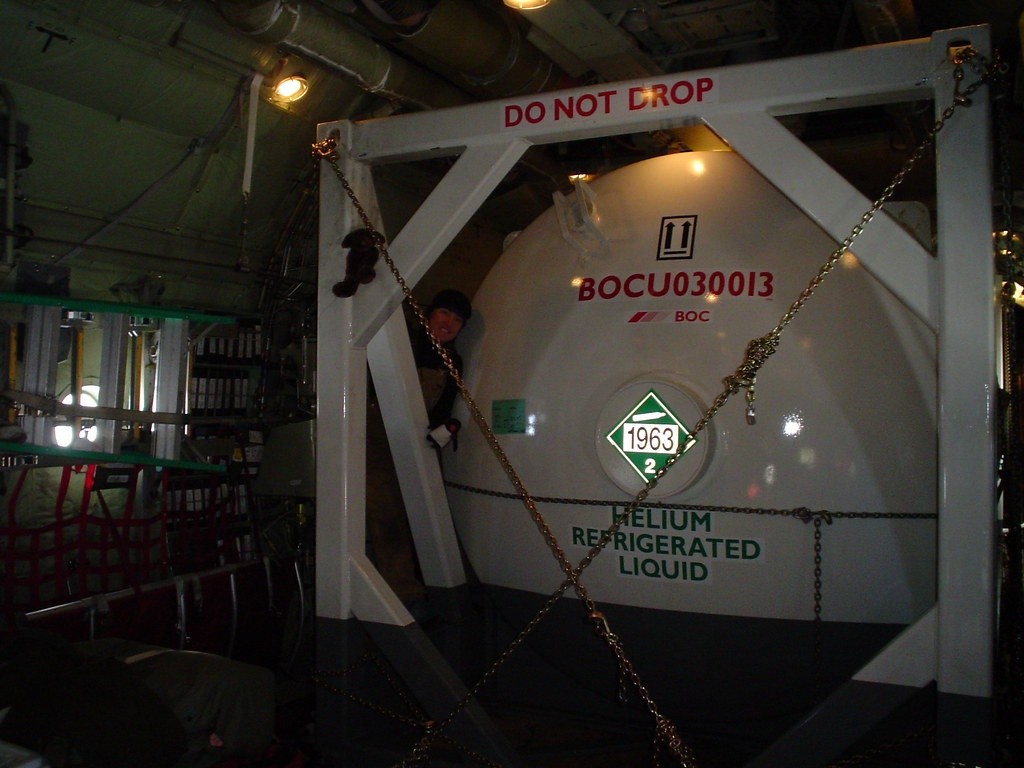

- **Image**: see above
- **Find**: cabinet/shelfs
[133,305,266,536]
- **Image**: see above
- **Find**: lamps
[623,9,649,33]
[273,74,309,103]
[504,0,550,10]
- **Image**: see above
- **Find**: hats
[433,290,472,319]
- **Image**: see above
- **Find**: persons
[409,285,470,452]
[3,634,278,767]
